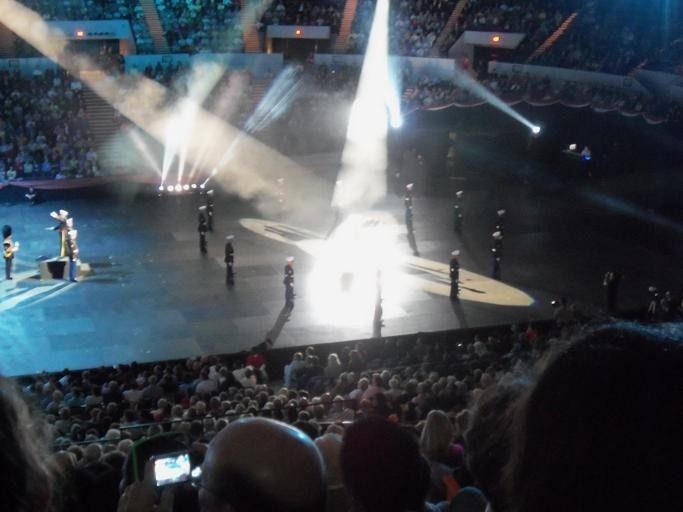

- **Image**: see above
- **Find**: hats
[404,183,505,215]
[285,255,294,261]
[1,207,78,240]
[207,189,213,195]
[450,249,460,256]
[492,230,501,238]
[198,205,208,211]
[225,234,235,240]
